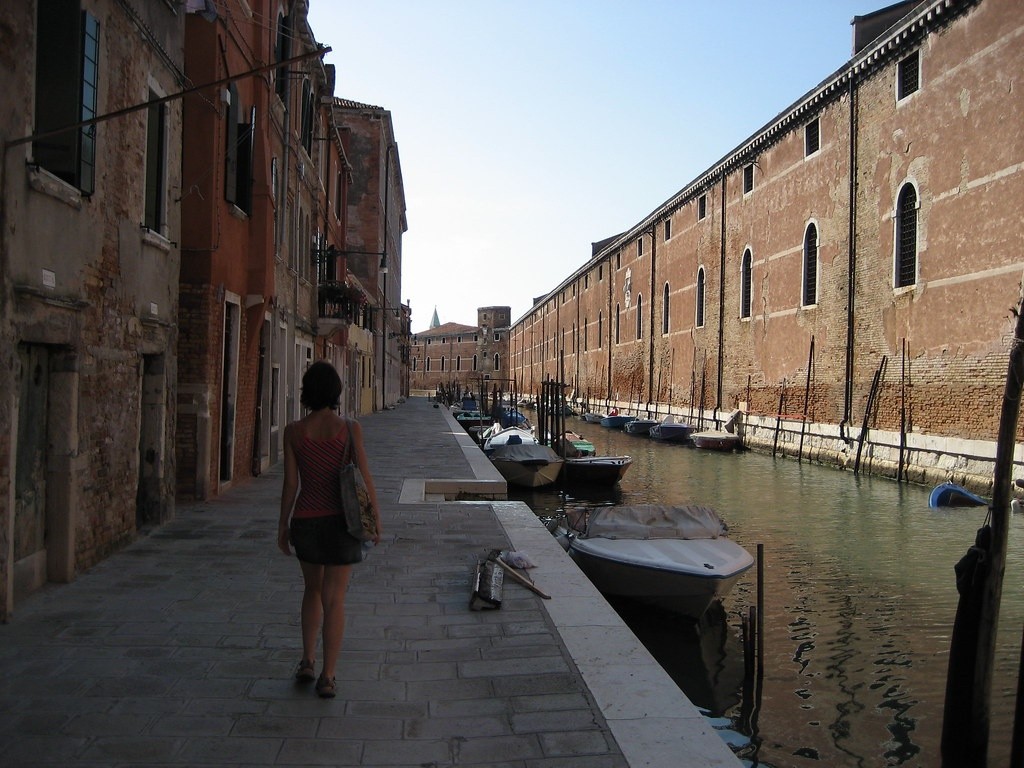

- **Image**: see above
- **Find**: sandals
[315,672,337,698]
[296,660,315,681]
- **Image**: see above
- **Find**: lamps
[394,307,400,323]
[378,254,388,273]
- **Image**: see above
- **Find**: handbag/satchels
[339,415,378,541]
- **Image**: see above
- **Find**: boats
[647,423,697,442]
[624,420,662,436]
[448,388,636,497]
[540,492,747,623]
[688,430,741,451]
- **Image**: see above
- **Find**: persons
[278,359,385,703]
